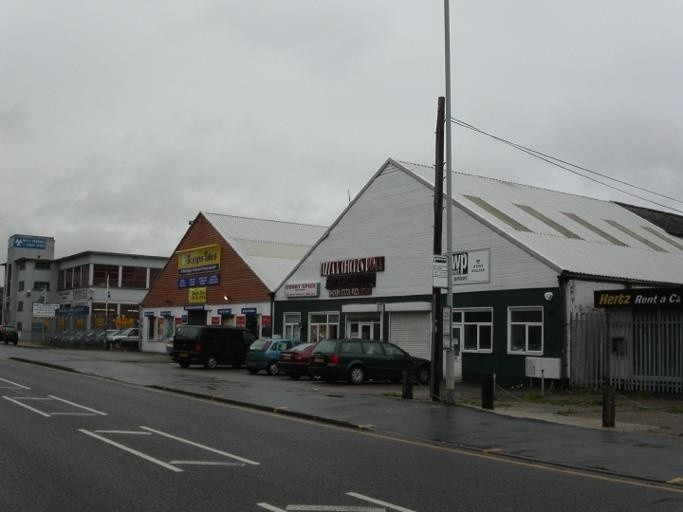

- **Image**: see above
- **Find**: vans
[167,326,257,369]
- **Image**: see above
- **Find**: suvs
[0,324,18,346]
[306,339,431,383]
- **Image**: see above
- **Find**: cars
[42,328,139,349]
[246,338,297,374]
[277,341,315,377]
